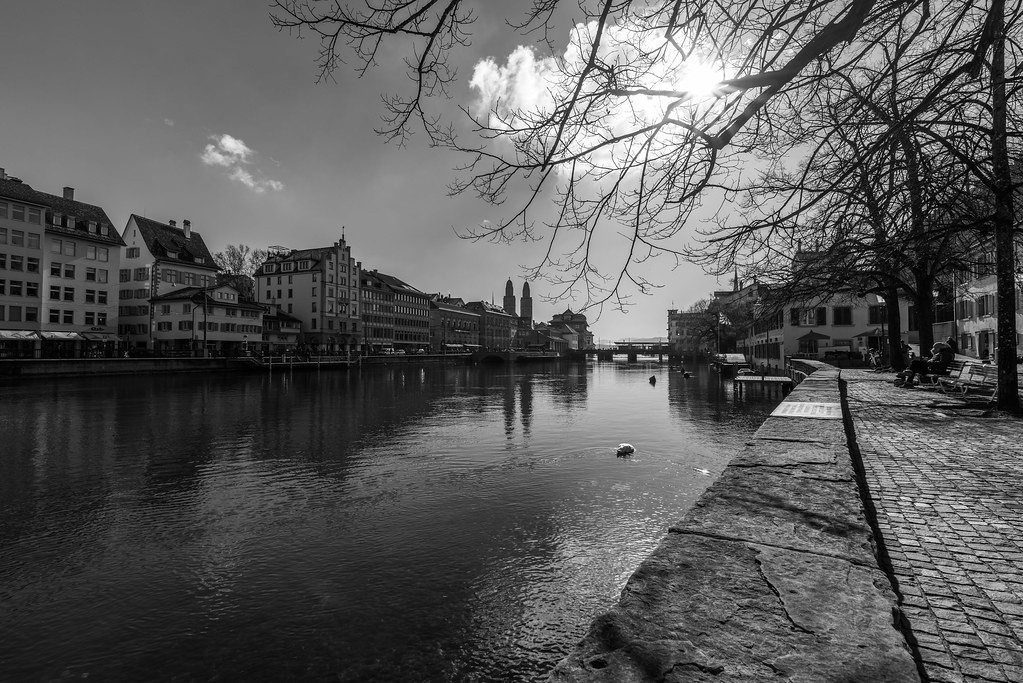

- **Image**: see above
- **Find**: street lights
[599,337,600,350]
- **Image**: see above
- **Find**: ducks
[616,442,634,457]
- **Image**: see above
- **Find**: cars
[379,348,394,355]
[412,349,425,355]
[394,349,406,355]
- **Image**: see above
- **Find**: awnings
[40,332,86,340]
[83,333,123,341]
[0,330,40,340]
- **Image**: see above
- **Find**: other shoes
[900,382,914,388]
[898,372,912,376]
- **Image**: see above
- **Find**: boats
[737,368,754,375]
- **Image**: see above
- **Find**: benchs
[926,361,968,389]
[937,363,999,405]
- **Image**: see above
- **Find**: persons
[899,343,952,388]
[947,338,958,358]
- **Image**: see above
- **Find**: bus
[464,344,483,353]
[443,344,464,353]
